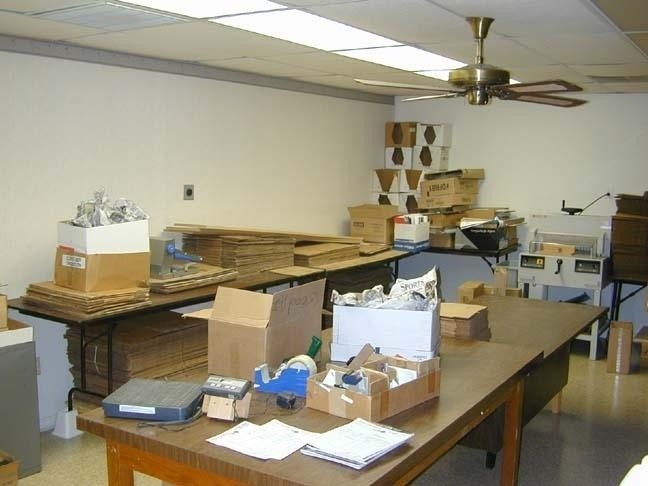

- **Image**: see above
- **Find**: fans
[354,16,588,107]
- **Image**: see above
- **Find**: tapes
[287,355,318,376]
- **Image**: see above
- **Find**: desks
[456,295,610,469]
[606,278,648,357]
[77,326,544,486]
[267,249,418,330]
[425,245,518,275]
[7,271,295,412]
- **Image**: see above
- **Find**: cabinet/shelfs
[0,341,42,480]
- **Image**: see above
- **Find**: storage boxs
[456,280,522,304]
[306,344,441,423]
[347,122,527,253]
[52,219,151,291]
[607,321,633,374]
[0,294,34,348]
[0,450,21,486]
[181,277,326,382]
[330,299,442,363]
